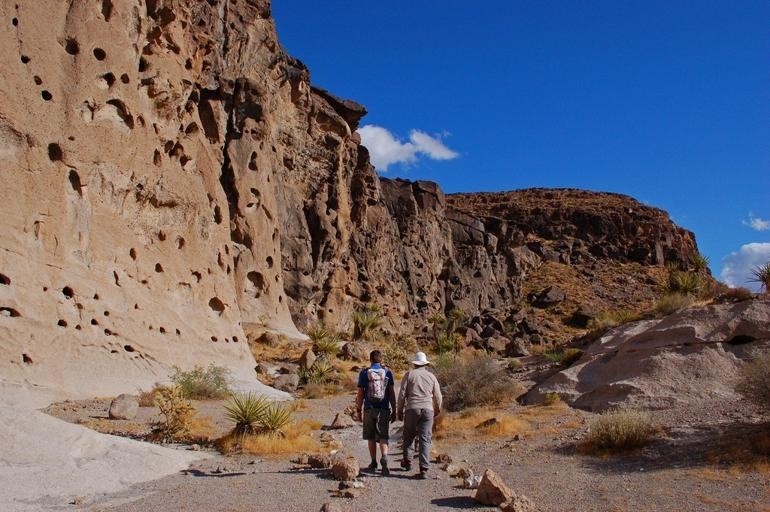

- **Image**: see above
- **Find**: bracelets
[356,408,361,413]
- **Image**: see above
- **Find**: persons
[356,350,397,477]
[398,351,443,479]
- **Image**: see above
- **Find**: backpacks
[367,369,388,401]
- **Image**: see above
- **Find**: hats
[408,352,429,365]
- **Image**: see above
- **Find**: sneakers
[380,457,389,476]
[422,469,429,479]
[401,460,412,469]
[369,461,377,467]
